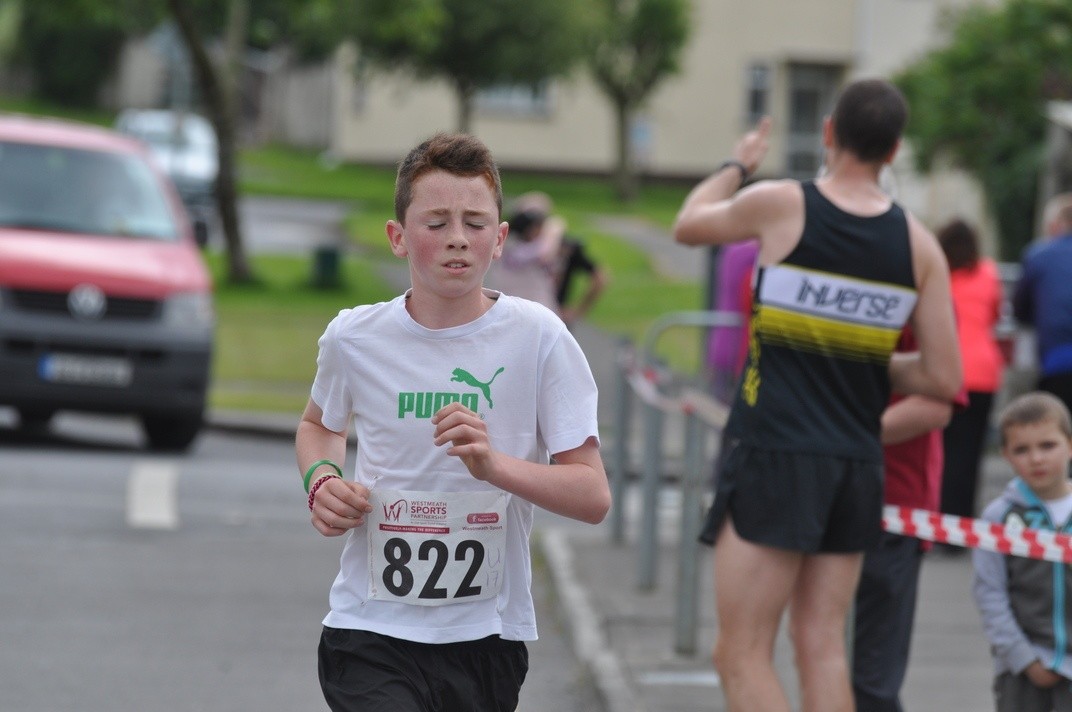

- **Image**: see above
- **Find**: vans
[0,111,215,454]
[116,107,219,222]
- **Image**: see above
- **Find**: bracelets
[311,472,342,490]
[722,159,749,186]
[308,475,340,512]
[303,459,344,493]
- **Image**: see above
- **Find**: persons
[675,78,962,712]
[704,181,1071,712]
[295,131,610,712]
